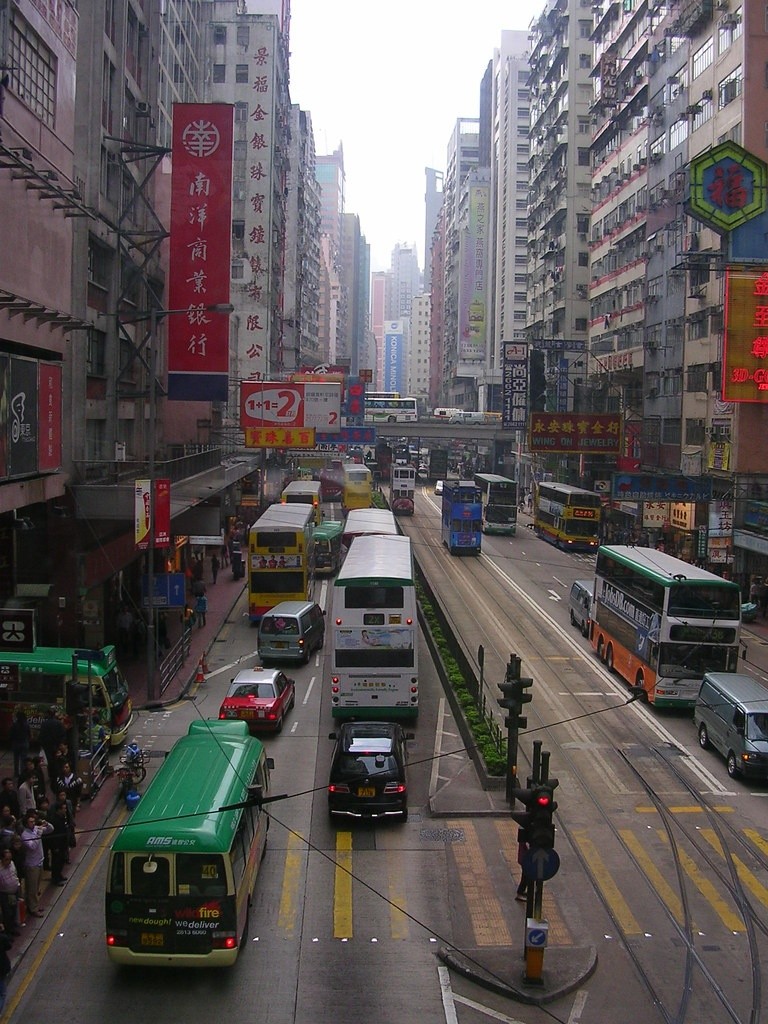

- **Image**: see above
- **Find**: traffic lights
[496,680,520,711]
[530,786,554,848]
[519,676,533,704]
[510,776,535,841]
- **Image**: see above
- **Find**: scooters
[117,742,147,793]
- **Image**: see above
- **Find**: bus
[441,480,485,555]
[310,521,344,576]
[429,448,448,480]
[388,462,416,503]
[281,481,326,527]
[364,397,419,423]
[364,391,401,407]
[339,509,398,572]
[330,534,420,723]
[247,503,317,625]
[534,482,602,552]
[473,473,521,534]
[588,544,748,710]
[320,459,342,503]
[340,463,373,515]
[105,718,276,970]
[0,643,134,748]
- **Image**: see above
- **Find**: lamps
[84,205,101,223]
[64,189,82,200]
[8,147,34,161]
[96,304,235,318]
[36,169,60,182]
[14,517,35,531]
[51,505,72,520]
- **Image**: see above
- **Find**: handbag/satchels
[39,747,48,766]
[68,824,76,847]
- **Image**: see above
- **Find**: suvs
[328,721,415,824]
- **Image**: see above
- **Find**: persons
[268,555,277,568]
[221,545,228,569]
[180,604,192,625]
[210,555,219,584]
[116,600,147,659]
[278,556,287,568]
[750,577,768,618]
[158,614,167,657]
[259,556,267,567]
[0,709,83,988]
[193,592,208,628]
[361,630,378,645]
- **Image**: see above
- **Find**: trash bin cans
[241,560,245,579]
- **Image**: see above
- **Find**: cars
[396,444,428,484]
[435,480,443,496]
[391,497,414,516]
[218,666,296,735]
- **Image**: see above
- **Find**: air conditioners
[650,187,662,204]
[622,174,631,180]
[614,180,622,186]
[587,206,651,258]
[602,176,610,182]
[669,173,685,190]
[611,278,642,296]
[592,275,598,281]
[639,159,647,164]
[611,167,619,173]
[594,183,602,189]
[577,284,588,292]
[644,341,658,349]
[690,286,700,296]
[654,245,664,252]
[597,260,604,266]
[704,427,730,435]
[633,164,640,170]
[645,294,655,302]
[215,27,226,36]
[136,101,151,118]
[652,153,661,160]
[658,189,670,199]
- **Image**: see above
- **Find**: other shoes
[32,911,43,917]
[38,907,44,911]
[61,876,67,881]
[15,922,26,927]
[57,882,65,887]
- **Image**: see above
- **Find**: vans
[449,412,486,425]
[434,408,464,419]
[256,601,327,665]
[693,671,768,780]
[568,579,595,638]
